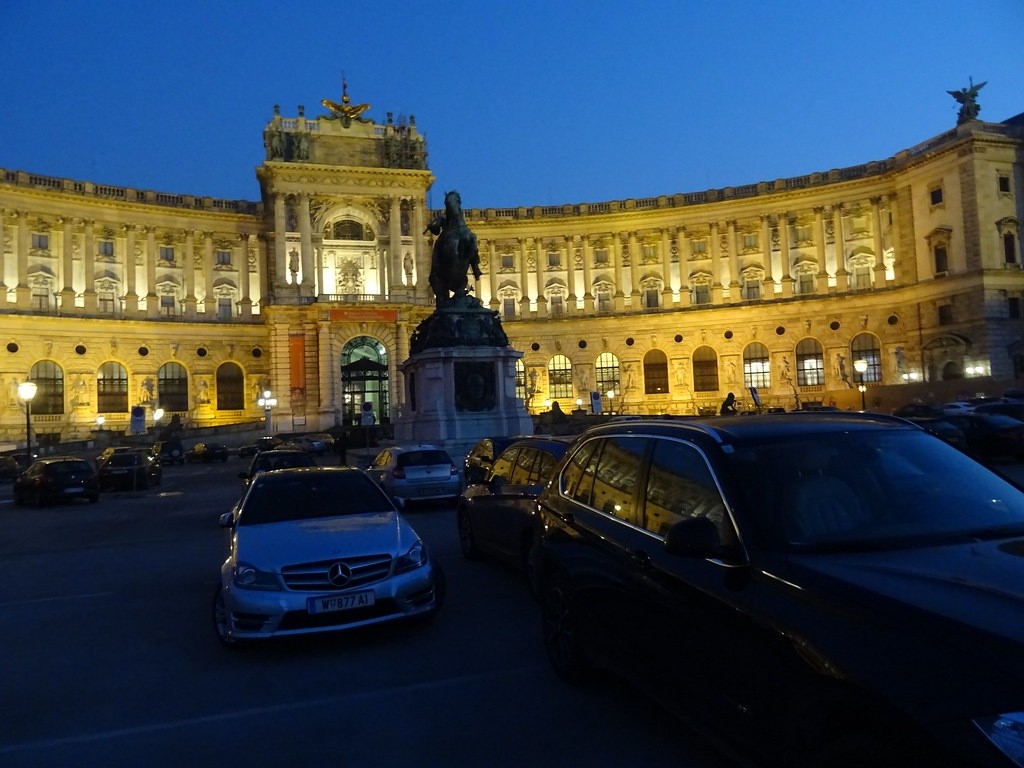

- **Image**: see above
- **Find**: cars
[213,464,440,652]
[97,452,161,492]
[13,459,99,508]
[369,443,465,514]
[890,389,1023,464]
[456,433,580,566]
[0,455,21,481]
[188,443,230,464]
[464,434,541,487]
[237,436,284,459]
[304,433,335,451]
[239,449,320,499]
[130,447,162,468]
[95,447,132,463]
[274,437,328,456]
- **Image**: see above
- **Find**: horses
[429,191,478,310]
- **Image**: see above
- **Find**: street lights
[853,358,868,411]
[20,381,38,466]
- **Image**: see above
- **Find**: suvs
[151,440,186,465]
[510,406,1024,768]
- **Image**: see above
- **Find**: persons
[721,393,737,417]
[947,76,987,115]
[427,210,482,280]
